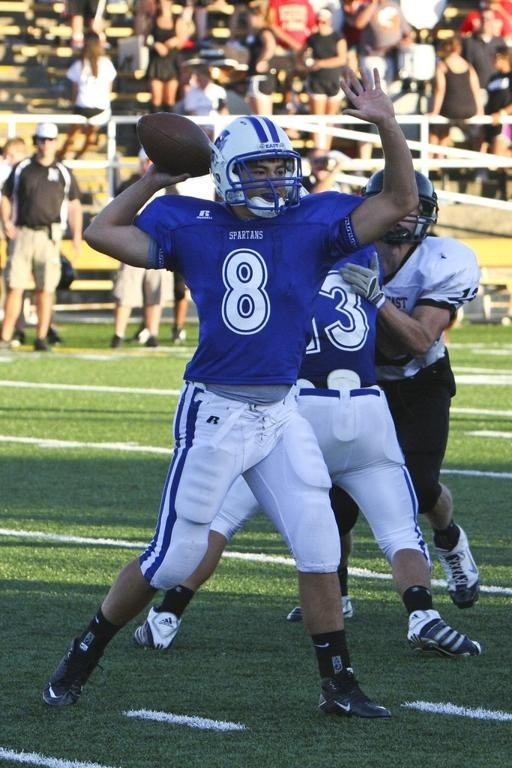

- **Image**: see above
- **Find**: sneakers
[134,606,183,650]
[285,594,355,621]
[318,668,391,718]
[111,326,187,348]
[43,637,103,707]
[433,521,479,609]
[406,609,481,659]
[1,327,63,354]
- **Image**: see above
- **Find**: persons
[0,0,512,160]
[108,148,213,348]
[278,162,483,624]
[37,61,422,722]
[131,236,483,662]
[0,122,84,353]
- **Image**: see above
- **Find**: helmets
[208,116,302,220]
[361,168,439,243]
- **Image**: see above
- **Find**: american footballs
[137,113,212,176]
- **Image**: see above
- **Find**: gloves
[340,251,385,310]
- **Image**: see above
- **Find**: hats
[35,122,59,141]
